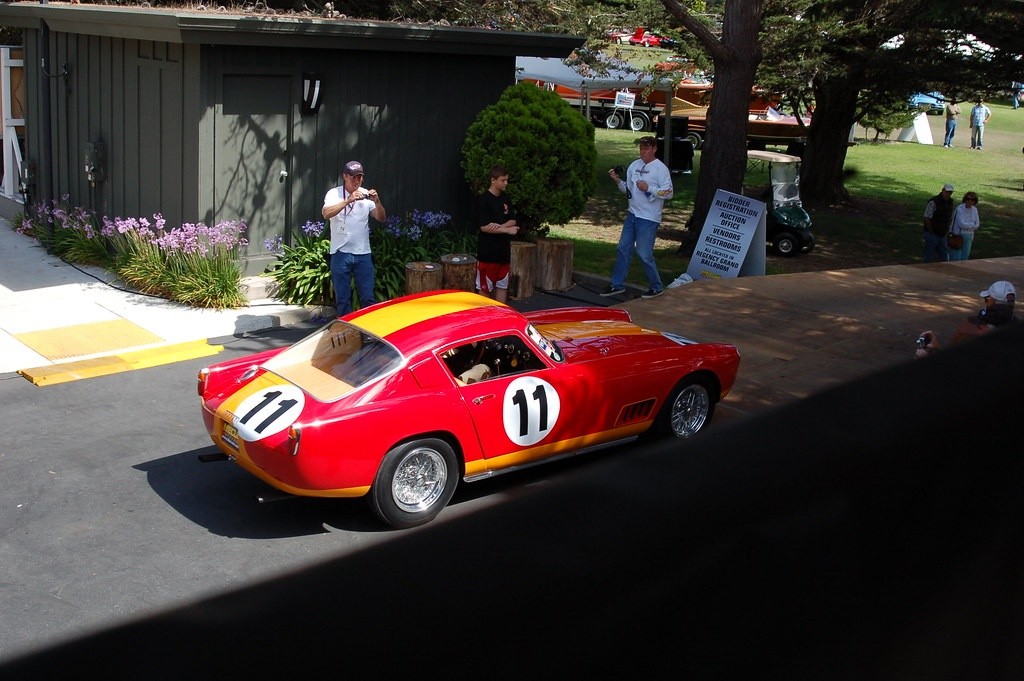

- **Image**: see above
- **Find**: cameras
[917,336,928,349]
[614,165,626,174]
[362,193,371,199]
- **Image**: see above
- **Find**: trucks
[516,56,782,131]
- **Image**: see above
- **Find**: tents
[515,49,675,123]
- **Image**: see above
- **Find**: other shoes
[943,144,954,148]
[969,147,976,150]
[978,146,983,150]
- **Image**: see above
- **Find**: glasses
[966,197,976,201]
[978,100,985,103]
[984,298,993,302]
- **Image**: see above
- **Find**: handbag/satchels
[947,232,964,250]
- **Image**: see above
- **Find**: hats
[634,136,658,147]
[978,305,1012,325]
[944,183,955,192]
[978,281,1018,303]
[343,161,364,179]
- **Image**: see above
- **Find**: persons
[322,162,385,317]
[1011,81,1019,108]
[981,281,1018,322]
[969,96,991,150]
[453,363,493,387]
[923,184,953,263]
[951,191,979,261]
[915,305,1012,358]
[600,136,674,299]
[943,96,961,149]
[476,165,520,305]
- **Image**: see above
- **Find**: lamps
[302,71,326,116]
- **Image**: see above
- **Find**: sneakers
[600,286,625,296]
[641,288,664,299]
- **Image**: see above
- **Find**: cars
[654,96,811,149]
[198,288,741,530]
[606,26,674,48]
[907,91,946,115]
